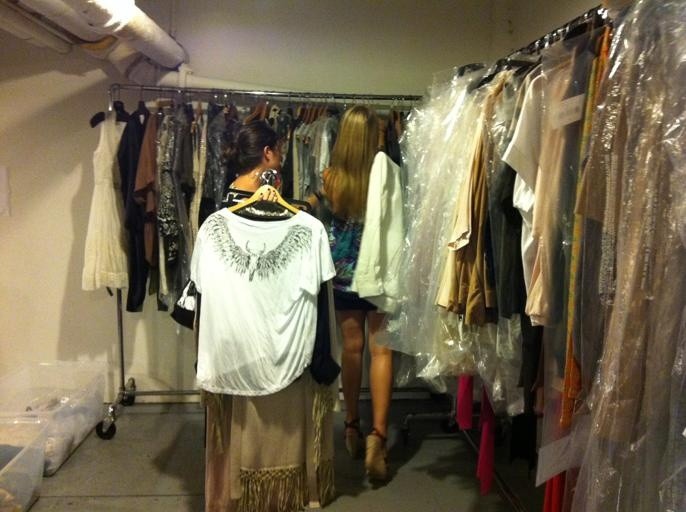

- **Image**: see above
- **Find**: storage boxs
[0,360,108,477]
[0,417,50,512]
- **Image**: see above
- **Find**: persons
[223,123,327,210]
[322,107,394,490]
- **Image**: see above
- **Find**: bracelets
[313,191,322,202]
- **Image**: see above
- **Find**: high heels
[366,427,389,482]
[343,417,362,461]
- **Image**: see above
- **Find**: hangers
[224,169,301,216]
[459,4,609,92]
